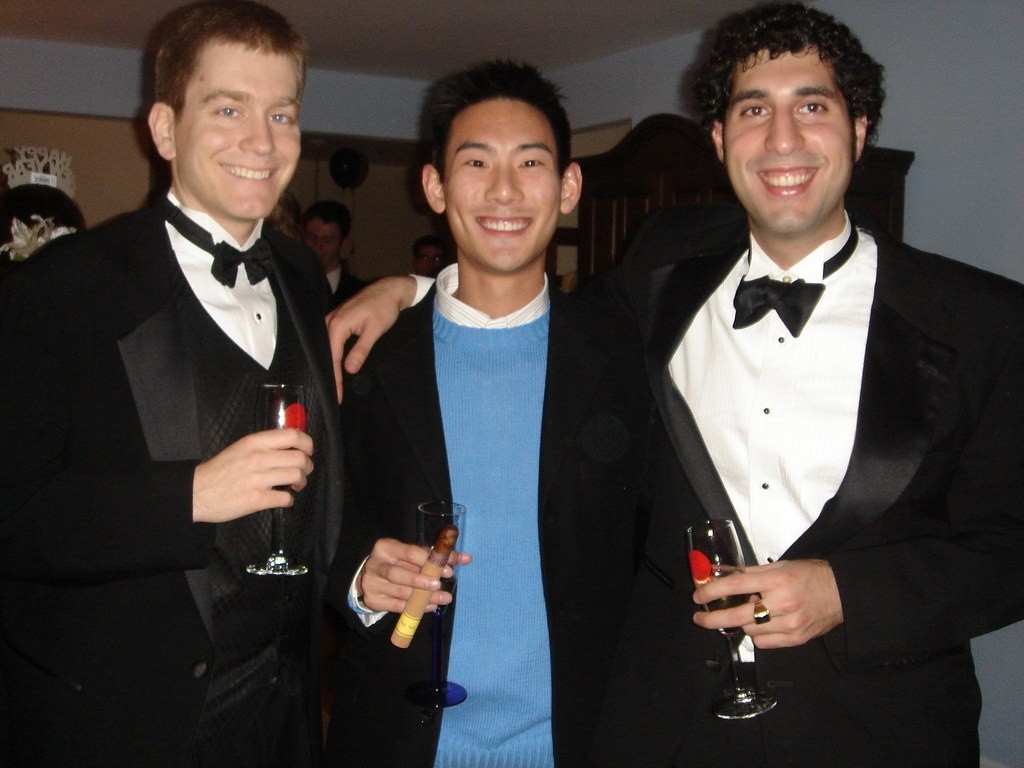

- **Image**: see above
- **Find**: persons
[303,199,369,303]
[0,0,348,768]
[268,196,315,254]
[323,54,669,768]
[2,183,86,265]
[410,228,446,283]
[324,0,1024,768]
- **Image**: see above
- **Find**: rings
[751,600,771,624]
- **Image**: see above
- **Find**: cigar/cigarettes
[389,523,461,649]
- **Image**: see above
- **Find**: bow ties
[211,239,274,289]
[730,273,826,337]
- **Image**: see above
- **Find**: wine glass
[243,383,307,575]
[687,517,779,717]
[405,501,467,707]
